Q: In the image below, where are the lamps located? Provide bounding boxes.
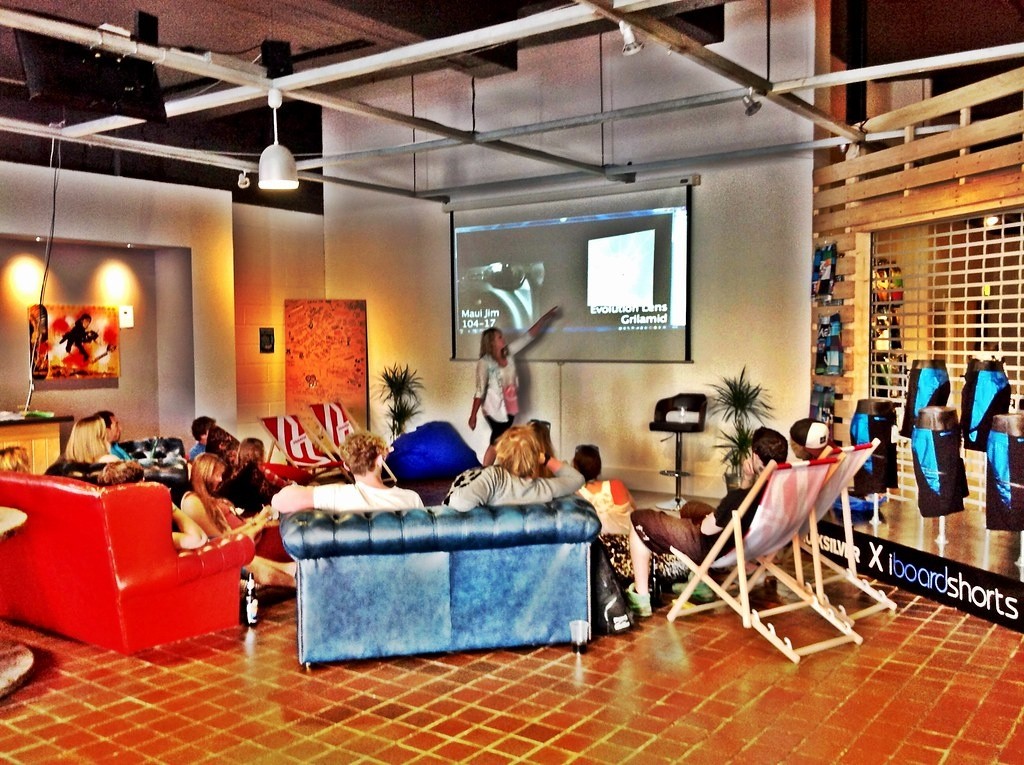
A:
[742,86,761,117]
[258,88,299,190]
[617,20,643,57]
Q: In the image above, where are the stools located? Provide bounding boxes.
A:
[649,392,708,511]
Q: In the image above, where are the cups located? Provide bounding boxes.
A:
[678,406,687,424]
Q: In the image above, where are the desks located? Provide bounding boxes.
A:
[0,505,35,697]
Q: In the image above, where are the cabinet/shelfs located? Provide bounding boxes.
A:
[0,415,75,476]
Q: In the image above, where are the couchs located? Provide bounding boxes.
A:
[280,494,602,671]
[0,470,255,657]
[117,437,190,511]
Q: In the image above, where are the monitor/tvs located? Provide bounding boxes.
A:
[10,7,169,126]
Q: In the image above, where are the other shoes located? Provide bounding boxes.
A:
[671,570,714,602]
[623,583,653,617]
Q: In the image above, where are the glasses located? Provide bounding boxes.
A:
[528,419,551,430]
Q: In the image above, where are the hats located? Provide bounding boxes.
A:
[789,418,840,457]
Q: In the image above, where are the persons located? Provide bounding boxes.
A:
[574,445,635,535]
[443,419,586,512]
[0,446,31,474]
[182,417,296,588]
[749,418,843,609]
[466,304,560,467]
[44,410,131,486]
[100,459,207,549]
[271,433,426,511]
[628,426,788,616]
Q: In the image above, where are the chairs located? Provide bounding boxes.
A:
[260,415,355,484]
[667,437,897,664]
[310,403,398,483]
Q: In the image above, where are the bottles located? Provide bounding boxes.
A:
[650,555,662,607]
[243,572,259,627]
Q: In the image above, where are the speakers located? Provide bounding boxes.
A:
[261,40,294,79]
[136,9,160,46]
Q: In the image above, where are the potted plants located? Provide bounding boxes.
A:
[706,365,776,495]
